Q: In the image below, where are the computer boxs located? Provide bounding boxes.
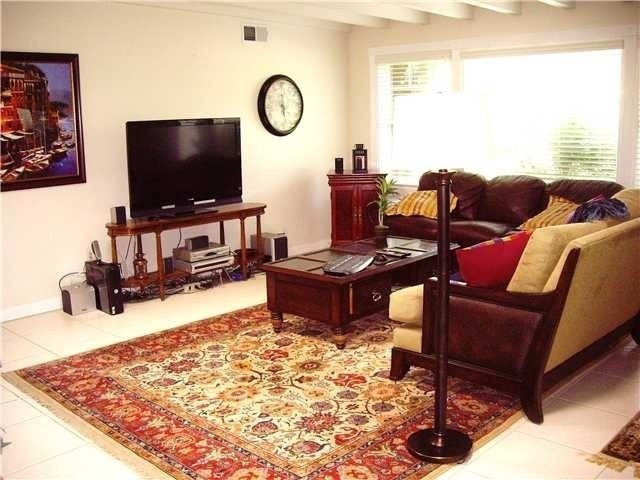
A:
[85,260,124,315]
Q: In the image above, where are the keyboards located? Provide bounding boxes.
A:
[323,254,375,276]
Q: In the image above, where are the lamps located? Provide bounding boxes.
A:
[390,93,489,464]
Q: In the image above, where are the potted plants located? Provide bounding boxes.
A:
[365,175,401,243]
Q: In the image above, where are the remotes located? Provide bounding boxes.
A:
[384,248,411,256]
[376,250,405,258]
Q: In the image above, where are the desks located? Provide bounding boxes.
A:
[105,204,267,300]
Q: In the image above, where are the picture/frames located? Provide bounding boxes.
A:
[0,51,86,191]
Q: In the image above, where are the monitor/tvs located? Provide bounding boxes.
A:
[126,117,243,218]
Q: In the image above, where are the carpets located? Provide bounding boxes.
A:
[586,409,640,480]
[0,304,632,479]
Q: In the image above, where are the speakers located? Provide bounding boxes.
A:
[91,240,102,264]
[249,231,288,262]
[111,207,126,224]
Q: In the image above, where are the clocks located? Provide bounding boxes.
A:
[257,75,303,136]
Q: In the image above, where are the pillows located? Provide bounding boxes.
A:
[604,187,640,227]
[543,179,624,210]
[419,170,487,220]
[482,175,549,225]
[516,195,580,232]
[455,230,531,286]
[387,189,458,219]
[506,221,608,295]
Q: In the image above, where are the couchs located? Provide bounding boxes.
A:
[390,217,640,425]
[383,211,549,248]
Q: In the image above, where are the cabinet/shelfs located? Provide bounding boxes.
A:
[327,174,388,249]
